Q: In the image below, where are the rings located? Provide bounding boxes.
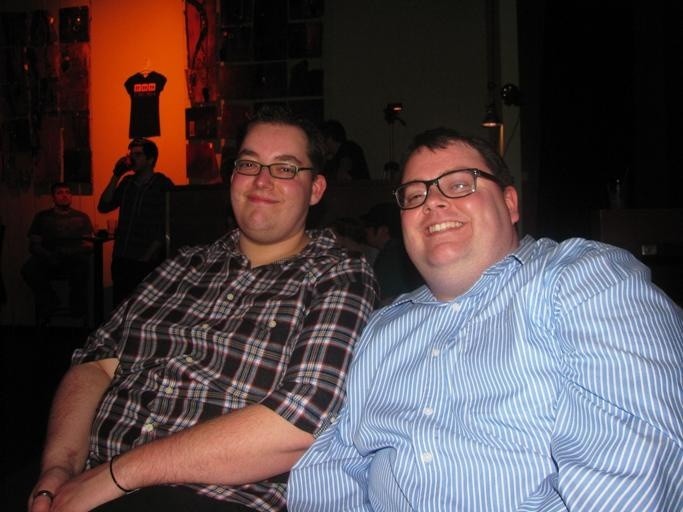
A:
[33,489,53,500]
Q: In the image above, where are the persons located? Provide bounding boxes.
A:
[20,182,92,307]
[316,121,423,306]
[96,138,173,307]
[286,126,682,511]
[27,106,381,511]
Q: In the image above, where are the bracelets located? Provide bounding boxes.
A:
[109,455,131,492]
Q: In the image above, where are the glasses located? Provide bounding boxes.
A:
[392,169,496,210]
[235,160,312,179]
